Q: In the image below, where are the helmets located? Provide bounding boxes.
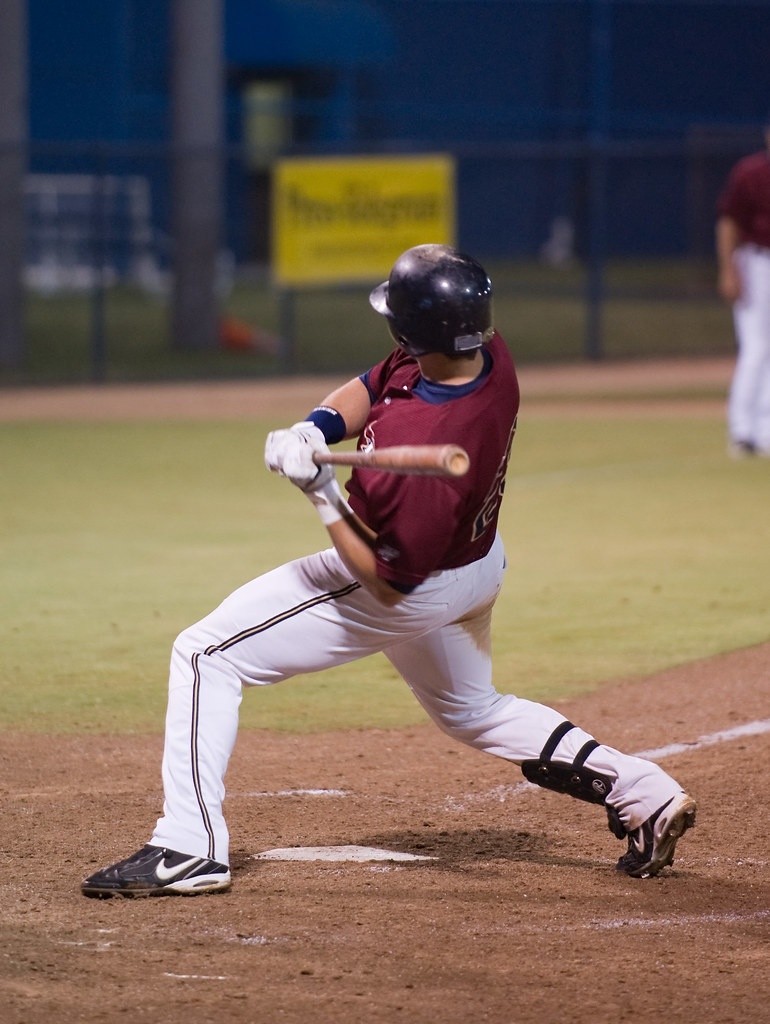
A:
[369,243,495,352]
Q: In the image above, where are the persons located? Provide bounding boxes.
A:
[80,245,700,897]
[718,126,770,460]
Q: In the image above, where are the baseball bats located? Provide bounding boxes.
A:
[313,442,472,478]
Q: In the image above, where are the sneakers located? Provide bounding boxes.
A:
[81,845,231,898]
[617,792,698,880]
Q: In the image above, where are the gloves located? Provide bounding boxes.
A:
[263,420,334,494]
